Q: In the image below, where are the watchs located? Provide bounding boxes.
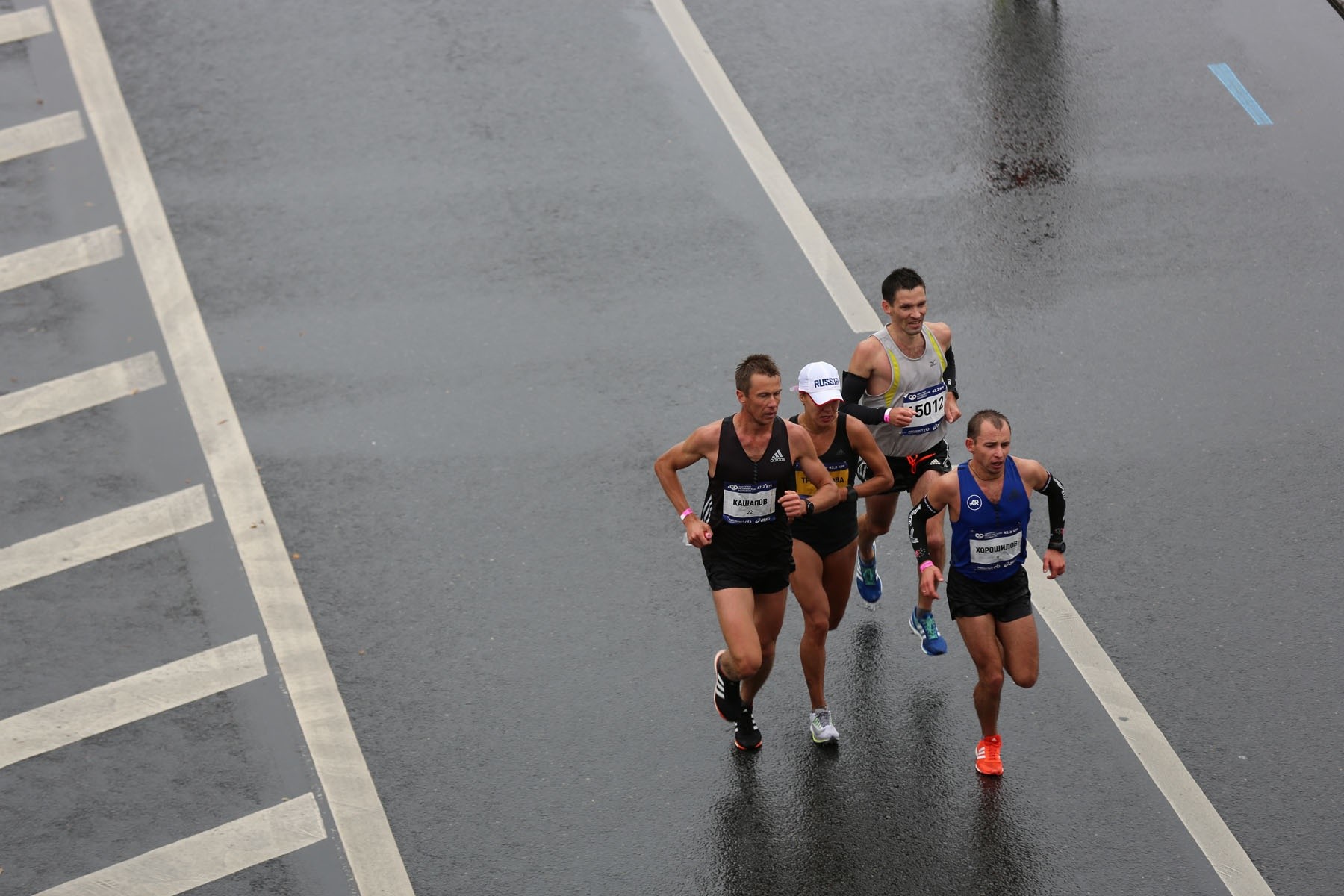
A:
[846,485,858,503]
[1047,542,1066,553]
[801,498,814,515]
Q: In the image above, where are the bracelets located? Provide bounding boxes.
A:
[884,407,892,424]
[919,561,934,574]
[680,508,692,521]
[948,387,959,400]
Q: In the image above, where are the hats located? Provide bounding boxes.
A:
[798,361,845,406]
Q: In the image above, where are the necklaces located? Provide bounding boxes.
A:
[967,461,1004,480]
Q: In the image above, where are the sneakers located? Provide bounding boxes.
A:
[809,705,840,744]
[908,606,947,656]
[733,700,762,750]
[975,735,1004,775]
[713,649,743,723]
[856,540,882,603]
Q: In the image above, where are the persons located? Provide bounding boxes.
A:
[837,266,962,657]
[786,362,895,744]
[908,408,1068,775]
[654,355,841,751]
